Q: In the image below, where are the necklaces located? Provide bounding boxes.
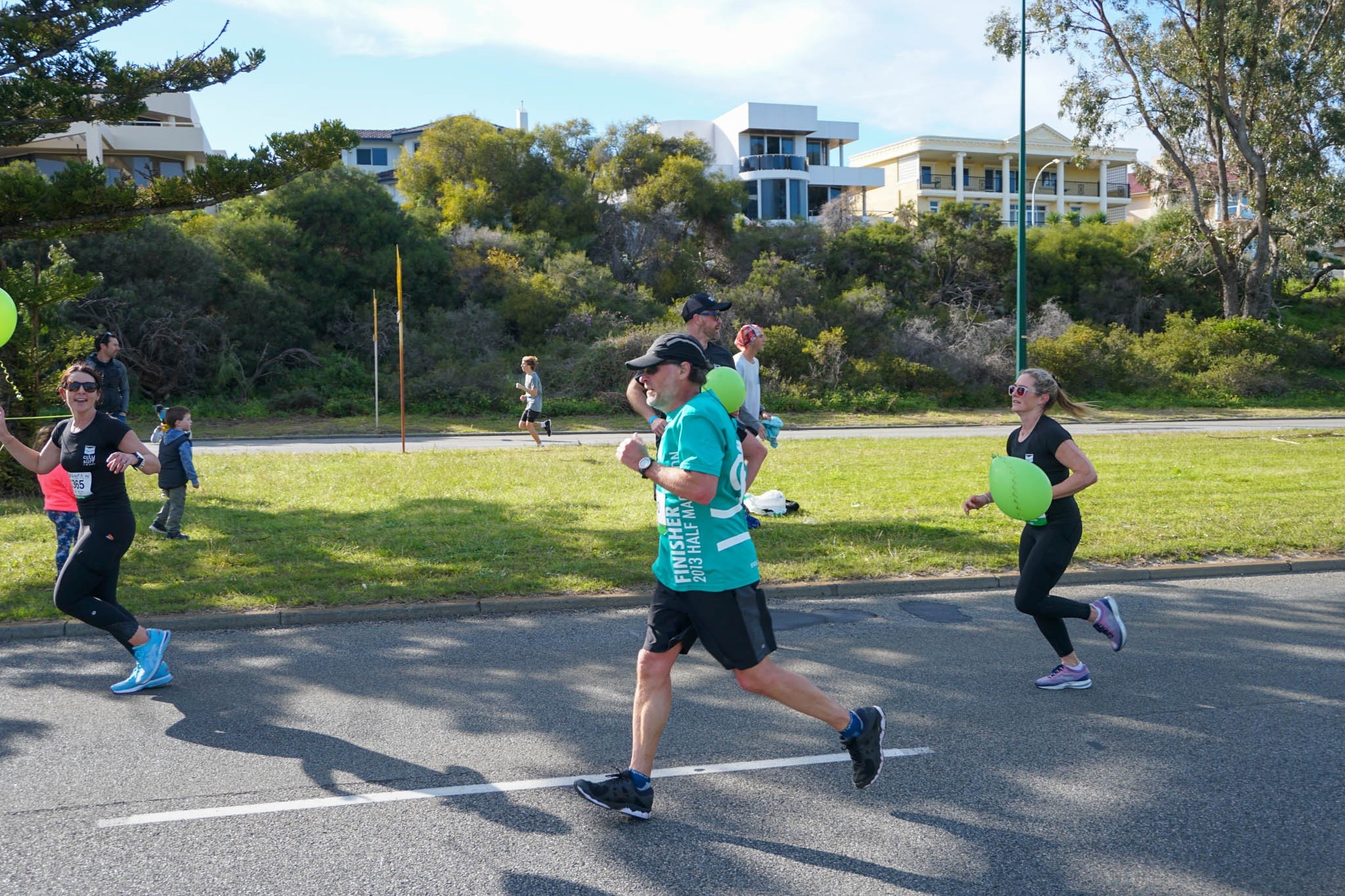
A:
[1021,414,1040,436]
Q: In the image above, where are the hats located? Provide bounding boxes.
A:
[733,323,764,351]
[625,332,714,371]
[682,293,733,323]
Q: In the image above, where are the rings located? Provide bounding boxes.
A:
[122,460,125,463]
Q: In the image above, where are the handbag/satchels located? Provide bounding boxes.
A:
[743,489,787,517]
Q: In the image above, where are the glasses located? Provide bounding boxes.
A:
[99,332,112,343]
[63,381,100,393]
[1008,385,1042,397]
[642,360,680,374]
[699,311,723,321]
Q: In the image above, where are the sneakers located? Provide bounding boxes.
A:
[572,764,654,820]
[109,660,174,694]
[543,419,552,437]
[131,628,171,685]
[166,532,190,540]
[148,521,168,534]
[838,705,888,791]
[741,503,761,529]
[1034,661,1092,690]
[1089,596,1128,652]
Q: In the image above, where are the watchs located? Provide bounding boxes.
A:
[638,456,655,479]
[131,451,146,471]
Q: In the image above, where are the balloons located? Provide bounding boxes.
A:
[0,288,17,347]
[701,366,746,414]
[989,453,1053,522]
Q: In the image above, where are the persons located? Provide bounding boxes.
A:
[0,362,173,694]
[573,333,887,820]
[31,424,80,580]
[515,355,552,448]
[625,293,772,531]
[85,331,129,423]
[963,368,1127,691]
[148,404,199,541]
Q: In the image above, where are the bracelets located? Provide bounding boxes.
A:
[982,494,990,507]
[648,415,658,426]
[3,436,15,445]
